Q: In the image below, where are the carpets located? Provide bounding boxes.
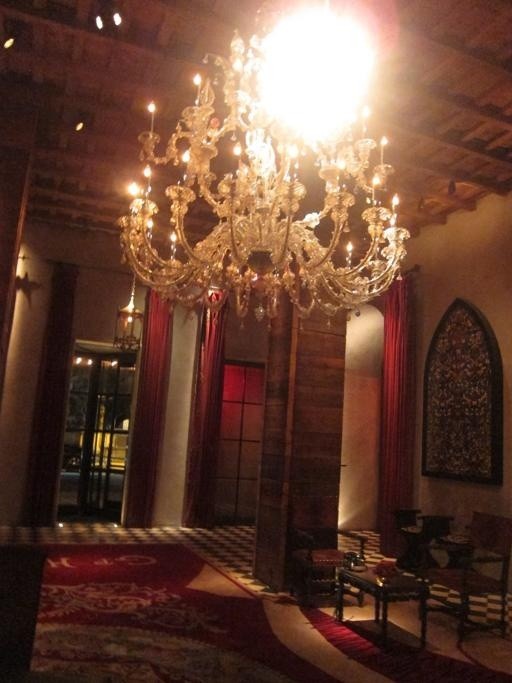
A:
[1,541,512,682]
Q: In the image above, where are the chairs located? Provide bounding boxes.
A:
[289,494,368,609]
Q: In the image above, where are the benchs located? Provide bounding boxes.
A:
[398,511,512,647]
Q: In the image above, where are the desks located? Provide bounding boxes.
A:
[337,565,429,648]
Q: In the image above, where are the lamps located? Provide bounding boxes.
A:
[117,1,410,335]
